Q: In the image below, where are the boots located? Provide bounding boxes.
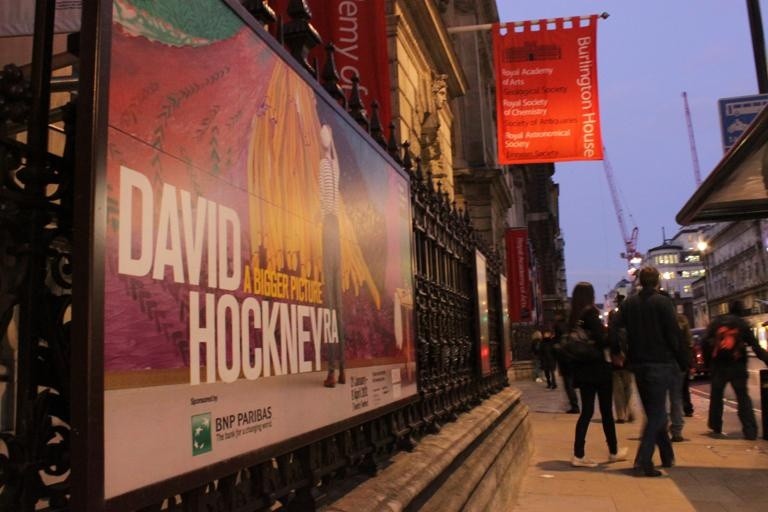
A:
[324,368,345,387]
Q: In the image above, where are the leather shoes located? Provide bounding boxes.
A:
[566,408,580,413]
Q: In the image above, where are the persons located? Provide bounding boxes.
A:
[532,328,559,388]
[699,301,767,440]
[313,121,348,389]
[608,267,694,472]
[553,314,580,413]
[569,282,628,467]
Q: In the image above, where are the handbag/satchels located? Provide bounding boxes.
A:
[551,323,591,365]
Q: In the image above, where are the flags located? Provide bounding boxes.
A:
[491,14,606,163]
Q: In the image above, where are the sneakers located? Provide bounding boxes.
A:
[635,460,675,476]
[608,447,628,461]
[570,456,598,467]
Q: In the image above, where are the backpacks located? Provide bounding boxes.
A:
[712,315,744,369]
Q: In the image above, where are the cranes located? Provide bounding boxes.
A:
[601,146,642,264]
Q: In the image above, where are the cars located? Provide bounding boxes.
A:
[689,327,710,380]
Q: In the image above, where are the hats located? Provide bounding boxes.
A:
[319,122,331,148]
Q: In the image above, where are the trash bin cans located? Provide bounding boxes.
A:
[758,369,767,441]
